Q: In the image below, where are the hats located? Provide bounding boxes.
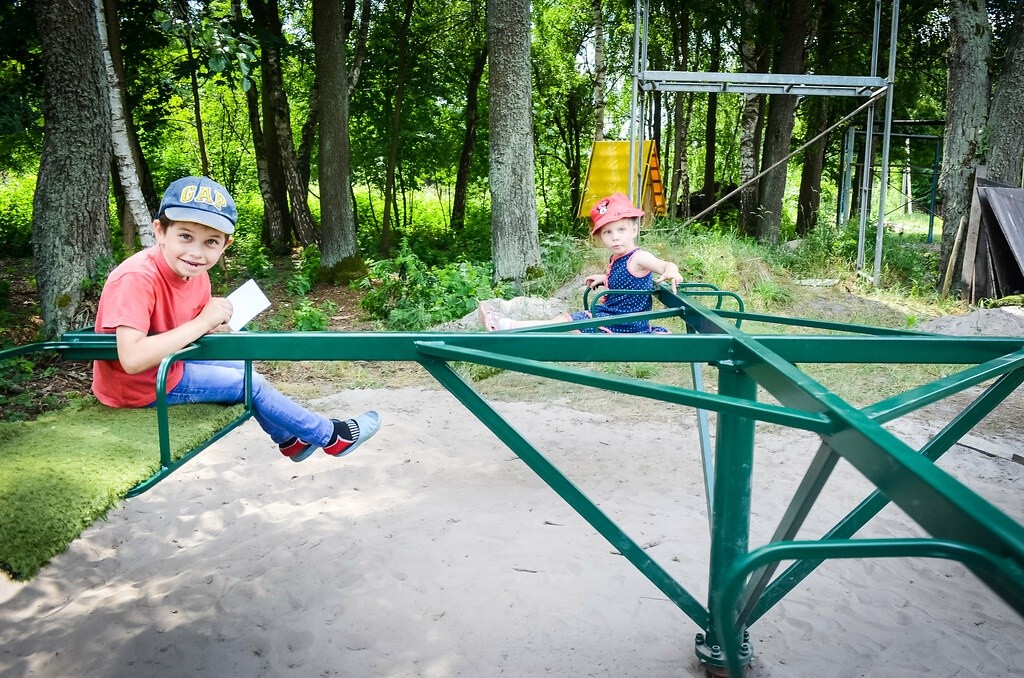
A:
[158,176,238,234]
[590,193,645,236]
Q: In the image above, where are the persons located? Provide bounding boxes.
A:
[478,191,682,335]
[91,176,381,462]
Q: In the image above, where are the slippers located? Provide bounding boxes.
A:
[289,419,340,463]
[333,411,381,458]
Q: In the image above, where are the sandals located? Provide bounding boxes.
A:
[478,306,501,332]
